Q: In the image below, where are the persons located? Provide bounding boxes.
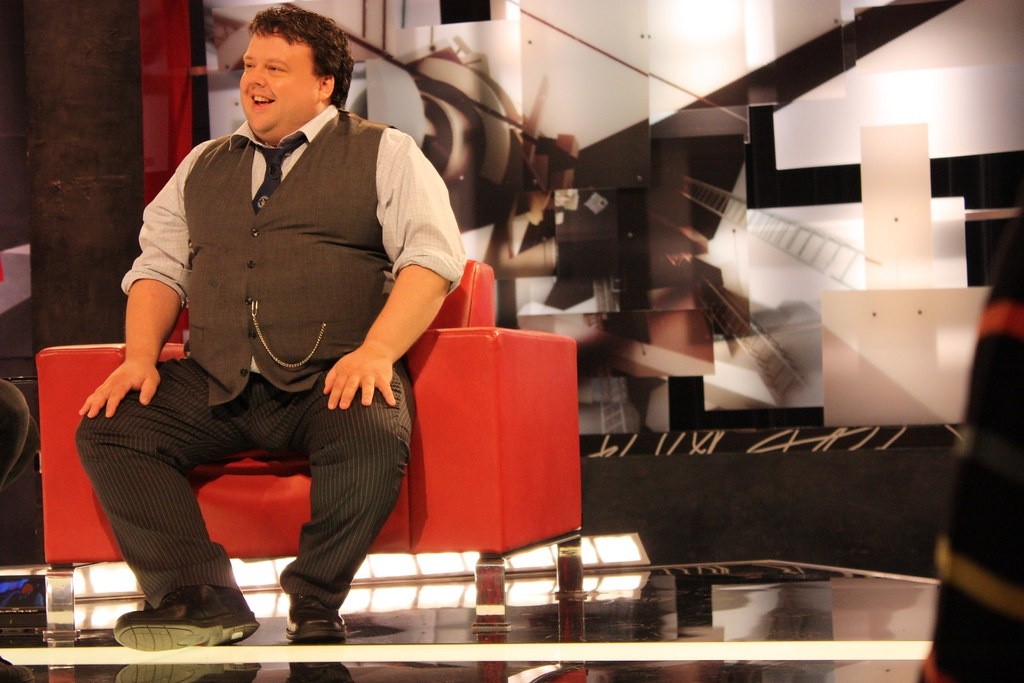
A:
[0,378,40,493]
[0,581,44,607]
[76,8,465,652]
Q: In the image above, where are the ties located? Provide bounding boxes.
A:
[249,133,311,216]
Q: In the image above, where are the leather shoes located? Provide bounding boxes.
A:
[285,586,347,642]
[113,582,261,652]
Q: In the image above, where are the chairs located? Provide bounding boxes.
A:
[35,257,583,639]
